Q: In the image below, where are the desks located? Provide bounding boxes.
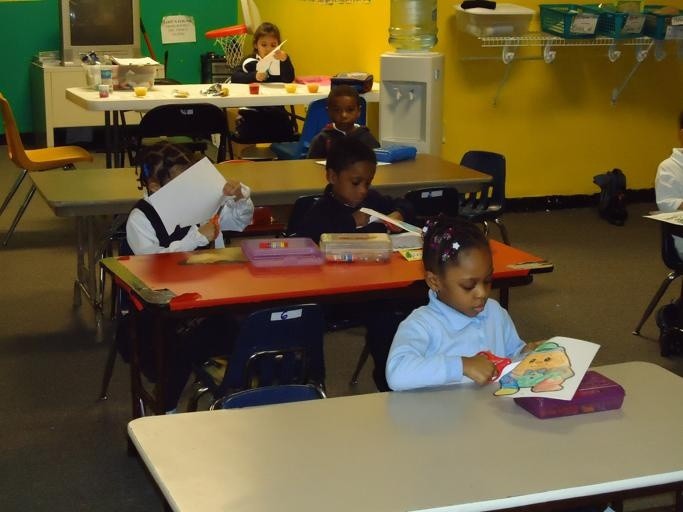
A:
[126,361,682,512]
[26,149,496,342]
[64,82,380,171]
[97,238,554,455]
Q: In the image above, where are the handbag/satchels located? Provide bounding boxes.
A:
[596,167,629,226]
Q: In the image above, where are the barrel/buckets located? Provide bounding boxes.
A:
[389,0,438,49]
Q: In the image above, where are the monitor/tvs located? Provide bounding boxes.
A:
[62,1,141,61]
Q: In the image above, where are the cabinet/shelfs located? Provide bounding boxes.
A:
[29,59,167,149]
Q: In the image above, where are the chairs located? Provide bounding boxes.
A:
[199,52,300,162]
[400,185,460,230]
[630,222,682,337]
[297,97,366,160]
[0,94,93,250]
[118,104,229,168]
[458,150,510,246]
[222,205,286,247]
[365,294,430,393]
[207,347,330,411]
[286,192,368,332]
[99,217,147,418]
[185,300,325,411]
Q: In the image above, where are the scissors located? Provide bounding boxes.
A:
[209,200,227,239]
[266,61,272,80]
[475,351,531,384]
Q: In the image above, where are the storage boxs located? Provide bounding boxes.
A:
[452,1,536,38]
[643,5,683,42]
[538,4,608,39]
[578,5,652,40]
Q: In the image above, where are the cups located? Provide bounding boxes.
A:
[248,84,259,95]
[132,86,149,97]
[305,84,320,93]
[98,84,113,98]
[284,84,297,94]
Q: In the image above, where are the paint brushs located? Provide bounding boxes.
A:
[259,241,289,248]
[337,235,377,239]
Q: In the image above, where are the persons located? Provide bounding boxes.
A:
[293,141,406,394]
[655,114,683,358]
[308,84,380,162]
[114,143,256,418]
[387,213,546,392]
[235,23,299,147]
